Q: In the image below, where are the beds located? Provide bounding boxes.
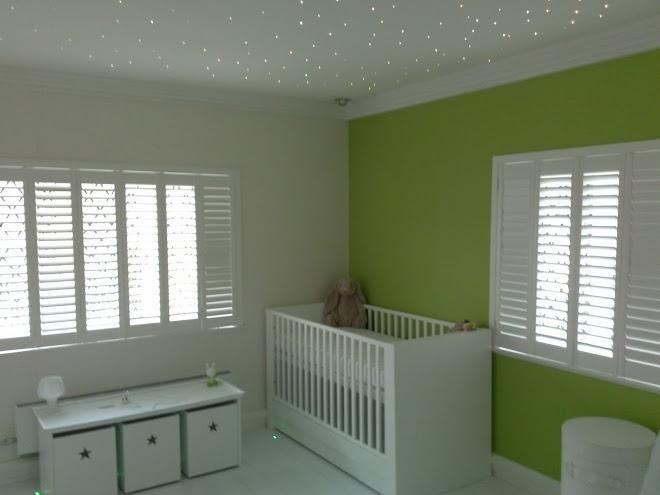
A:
[264,302,493,495]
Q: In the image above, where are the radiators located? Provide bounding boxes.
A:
[15,371,234,457]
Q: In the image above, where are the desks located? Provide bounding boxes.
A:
[32,370,246,495]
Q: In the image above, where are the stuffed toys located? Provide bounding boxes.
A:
[325,277,367,328]
